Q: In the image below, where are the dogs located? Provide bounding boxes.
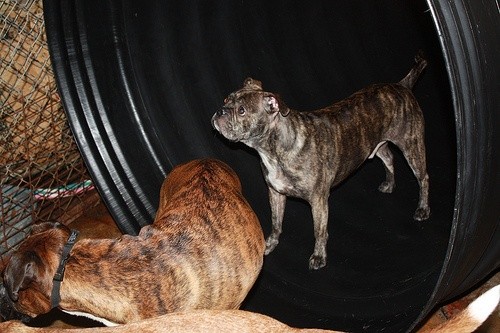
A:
[209,50,432,270]
[0,156,264,326]
[0,283,499,333]
